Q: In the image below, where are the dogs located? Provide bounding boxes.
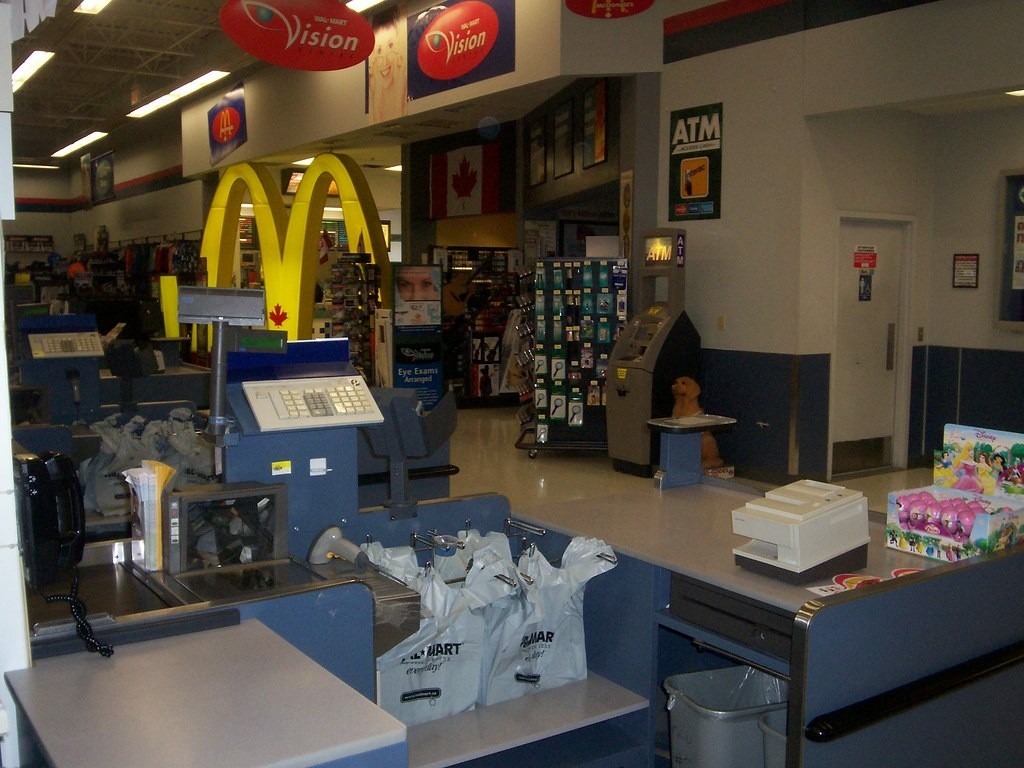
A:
[670,376,724,467]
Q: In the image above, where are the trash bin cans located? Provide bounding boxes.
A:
[664,664,788,768]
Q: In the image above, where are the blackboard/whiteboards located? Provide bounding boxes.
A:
[991,167,1024,330]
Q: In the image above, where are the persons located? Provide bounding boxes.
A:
[396,266,437,301]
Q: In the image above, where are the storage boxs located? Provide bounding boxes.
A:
[467,304,519,398]
[884,423,1023,563]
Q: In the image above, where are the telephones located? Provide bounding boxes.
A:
[27,450,86,586]
